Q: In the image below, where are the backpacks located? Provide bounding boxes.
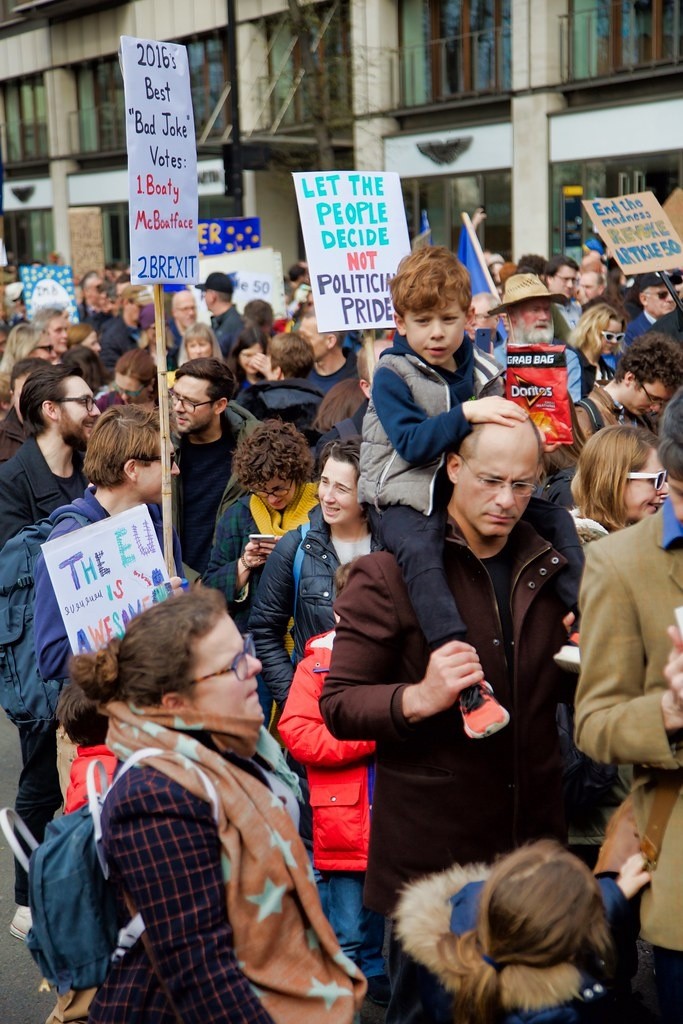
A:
[0,512,94,722]
[0,747,220,996]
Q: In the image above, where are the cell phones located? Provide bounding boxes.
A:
[248,533,275,546]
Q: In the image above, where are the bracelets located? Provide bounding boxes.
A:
[241,554,251,569]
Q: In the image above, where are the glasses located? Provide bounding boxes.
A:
[56,396,98,411]
[168,388,216,414]
[627,469,668,490]
[456,454,537,497]
[643,290,680,299]
[255,475,294,498]
[185,632,257,685]
[33,345,53,354]
[132,452,175,469]
[635,374,669,407]
[600,331,625,343]
[555,274,579,285]
[112,380,145,399]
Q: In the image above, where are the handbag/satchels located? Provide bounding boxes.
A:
[592,793,660,876]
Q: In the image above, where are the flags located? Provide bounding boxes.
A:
[458,226,508,345]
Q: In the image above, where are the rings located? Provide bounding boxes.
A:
[258,555,260,562]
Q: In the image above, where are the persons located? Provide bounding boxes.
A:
[0,246,683,1024]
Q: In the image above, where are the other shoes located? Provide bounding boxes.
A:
[10,914,30,940]
[366,974,392,1006]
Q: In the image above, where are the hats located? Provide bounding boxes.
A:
[195,272,234,294]
[635,271,683,290]
[487,273,568,315]
[5,282,24,300]
[140,303,169,328]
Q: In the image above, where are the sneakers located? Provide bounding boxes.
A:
[553,633,583,673]
[459,680,510,738]
